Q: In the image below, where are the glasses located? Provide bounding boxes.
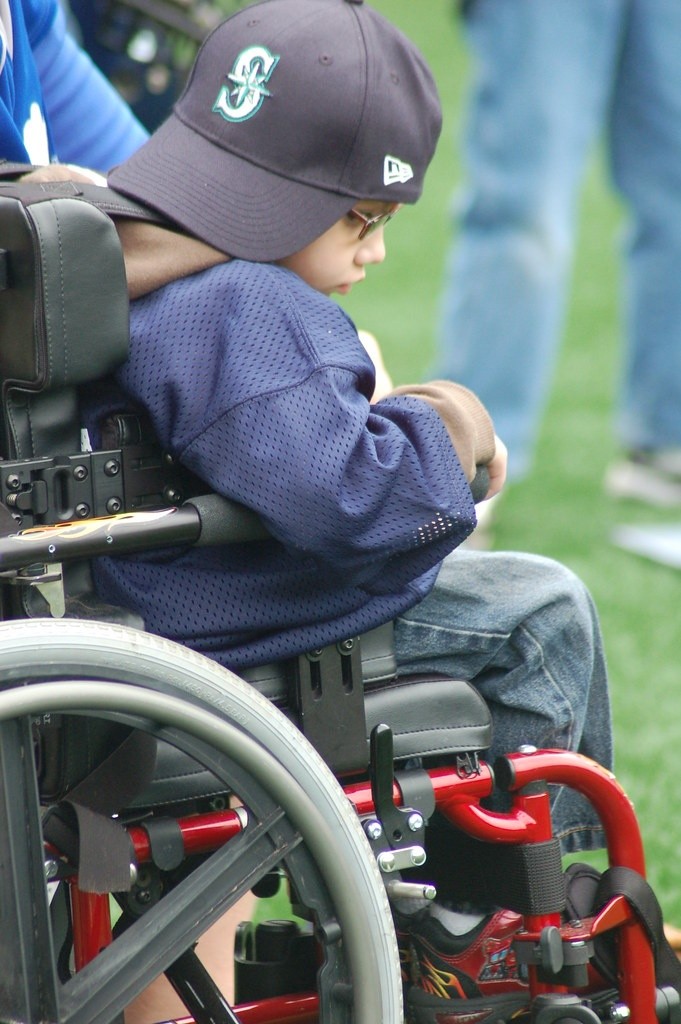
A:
[350,203,402,241]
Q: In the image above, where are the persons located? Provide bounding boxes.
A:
[431,0,681,524]
[0,0,615,1023]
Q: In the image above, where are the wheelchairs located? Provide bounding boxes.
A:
[1,184,681,1023]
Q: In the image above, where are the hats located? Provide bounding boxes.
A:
[106,0,443,262]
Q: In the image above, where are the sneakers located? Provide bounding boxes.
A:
[394,903,622,1024]
